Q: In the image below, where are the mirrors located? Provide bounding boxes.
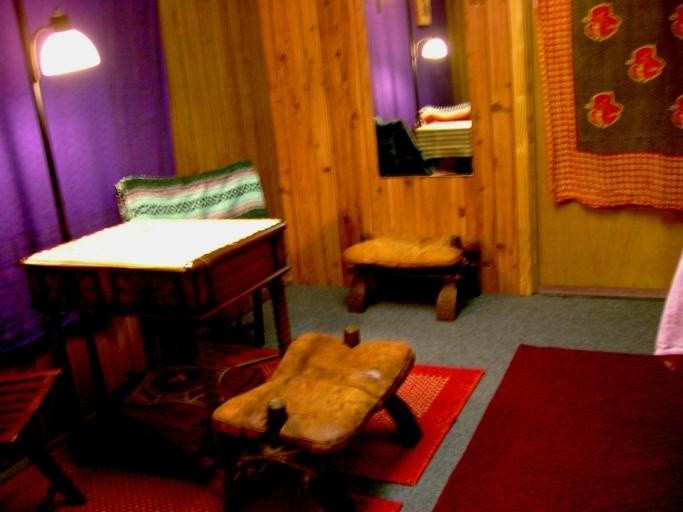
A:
[366,0,471,178]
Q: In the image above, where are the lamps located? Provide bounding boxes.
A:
[404,0,450,124]
[13,0,109,399]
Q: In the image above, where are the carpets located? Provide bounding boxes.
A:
[0,442,404,512]
[431,344,683,512]
[195,342,486,489]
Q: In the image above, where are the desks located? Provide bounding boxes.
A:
[21,216,292,395]
[414,120,472,173]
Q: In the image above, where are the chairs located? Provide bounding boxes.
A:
[113,160,270,347]
[375,115,432,177]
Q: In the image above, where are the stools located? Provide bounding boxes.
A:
[0,368,87,505]
[212,324,424,512]
[341,231,481,321]
